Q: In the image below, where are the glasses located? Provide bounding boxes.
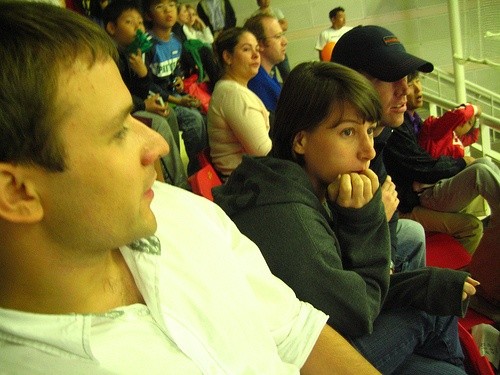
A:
[261,33,286,39]
[149,3,178,11]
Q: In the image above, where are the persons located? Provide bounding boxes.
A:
[413,104,499,212]
[0,0,386,375]
[329,24,434,273]
[242,13,287,112]
[315,7,355,62]
[211,61,480,375]
[248,0,291,85]
[383,68,485,257]
[88,1,237,191]
[206,27,272,185]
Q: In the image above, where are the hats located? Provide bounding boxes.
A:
[329,25,434,83]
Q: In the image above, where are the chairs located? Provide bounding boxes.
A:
[426,231,500,375]
